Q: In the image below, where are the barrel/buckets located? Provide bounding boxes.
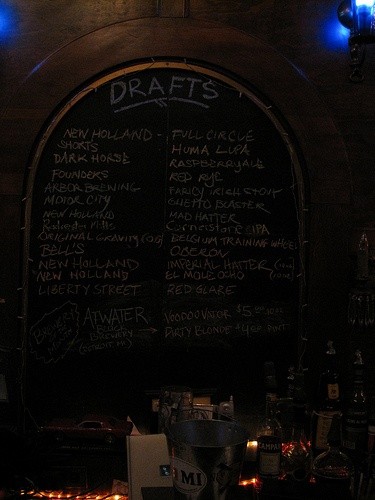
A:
[158,385,193,433]
[164,408,251,500]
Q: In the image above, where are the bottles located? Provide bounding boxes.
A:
[257,392,282,480]
[347,231,375,330]
[283,339,375,500]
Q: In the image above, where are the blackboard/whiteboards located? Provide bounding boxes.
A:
[21,63,305,433]
